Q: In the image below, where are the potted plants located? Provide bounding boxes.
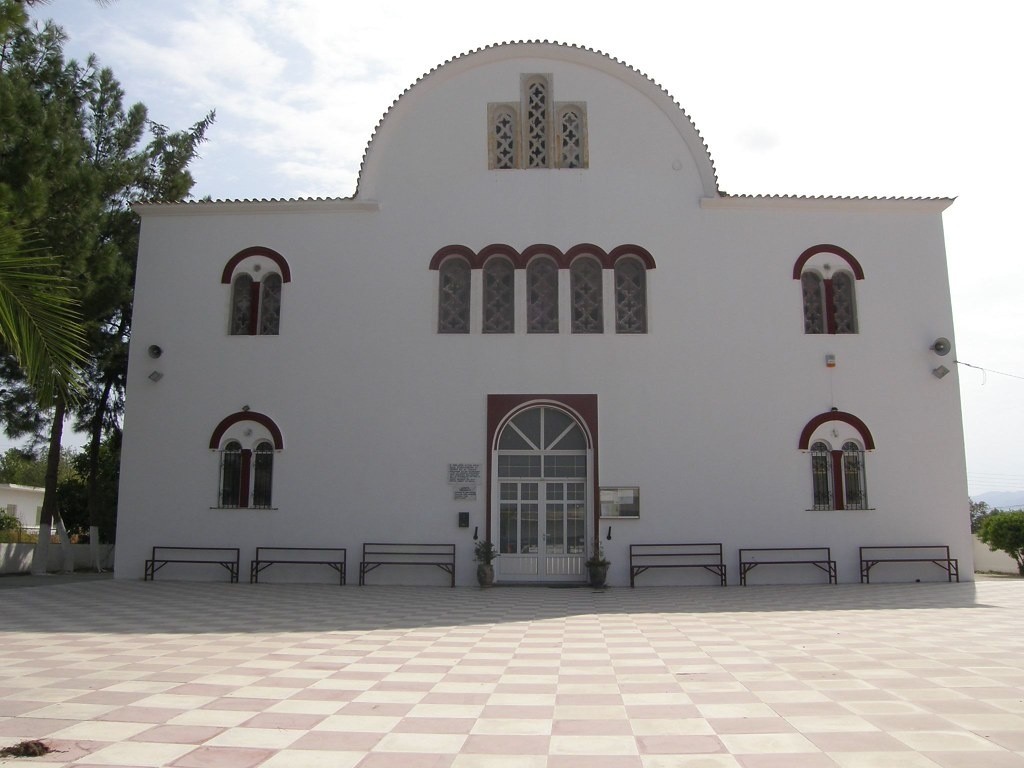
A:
[472,537,501,587]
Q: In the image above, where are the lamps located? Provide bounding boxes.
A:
[932,364,948,378]
[149,371,163,382]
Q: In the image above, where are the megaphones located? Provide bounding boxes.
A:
[148,344,163,359]
[929,337,952,356]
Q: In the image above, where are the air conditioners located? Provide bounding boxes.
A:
[584,536,611,585]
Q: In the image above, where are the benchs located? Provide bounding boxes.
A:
[629,543,727,588]
[859,546,959,583]
[144,546,240,582]
[251,547,346,585]
[739,548,838,586]
[359,542,456,588]
